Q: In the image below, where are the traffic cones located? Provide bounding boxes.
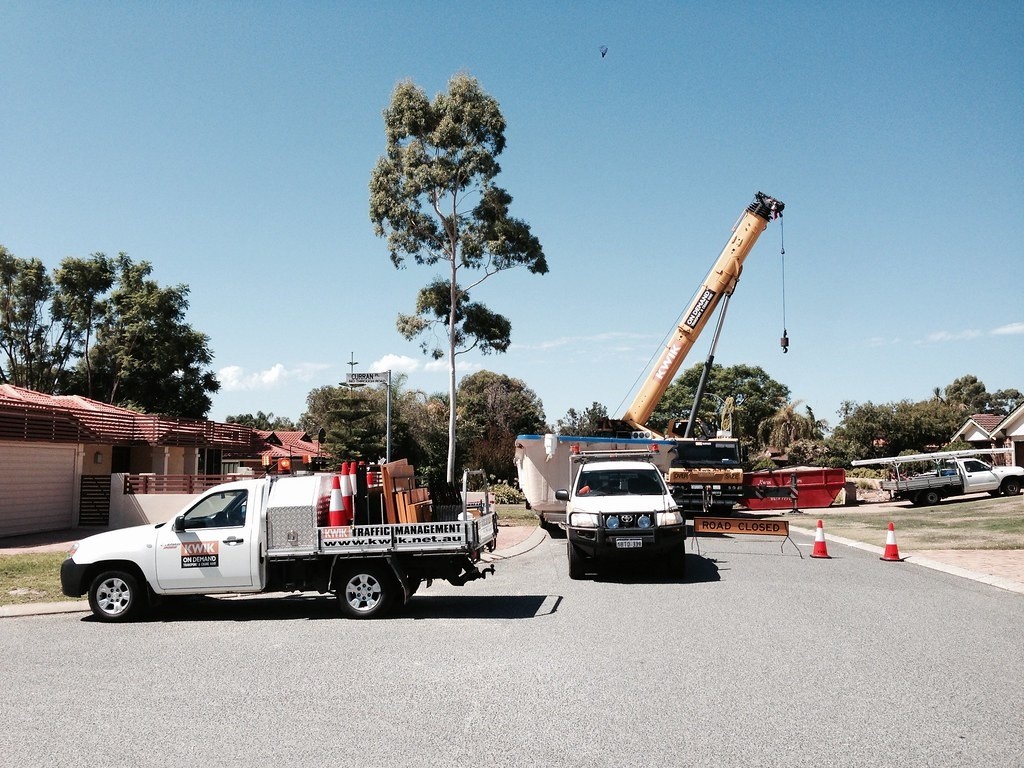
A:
[327,460,373,526]
[809,519,834,559]
[879,521,904,562]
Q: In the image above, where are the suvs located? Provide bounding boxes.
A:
[555,444,689,581]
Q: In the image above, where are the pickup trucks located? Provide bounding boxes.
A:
[880,458,1024,505]
[60,453,500,621]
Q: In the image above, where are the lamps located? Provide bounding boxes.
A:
[94,452,104,464]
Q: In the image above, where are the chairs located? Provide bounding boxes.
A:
[628,478,649,494]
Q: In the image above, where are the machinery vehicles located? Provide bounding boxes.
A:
[592,191,790,529]
